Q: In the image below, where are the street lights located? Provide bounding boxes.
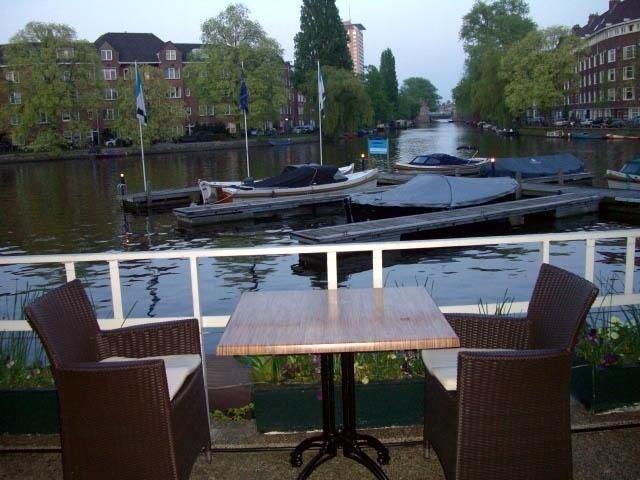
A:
[284,117,289,136]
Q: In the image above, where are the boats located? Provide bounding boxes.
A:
[545,130,640,140]
[448,120,454,123]
[465,119,520,138]
[357,119,416,138]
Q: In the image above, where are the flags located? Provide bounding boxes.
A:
[132,64,149,127]
[236,68,251,113]
[317,72,331,119]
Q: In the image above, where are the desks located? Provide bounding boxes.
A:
[214,283,463,478]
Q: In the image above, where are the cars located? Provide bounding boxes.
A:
[21,142,34,152]
[523,115,640,129]
[0,141,19,153]
[103,135,133,148]
[240,124,316,137]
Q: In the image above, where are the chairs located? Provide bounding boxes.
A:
[22,277,213,480]
[419,264,599,479]
